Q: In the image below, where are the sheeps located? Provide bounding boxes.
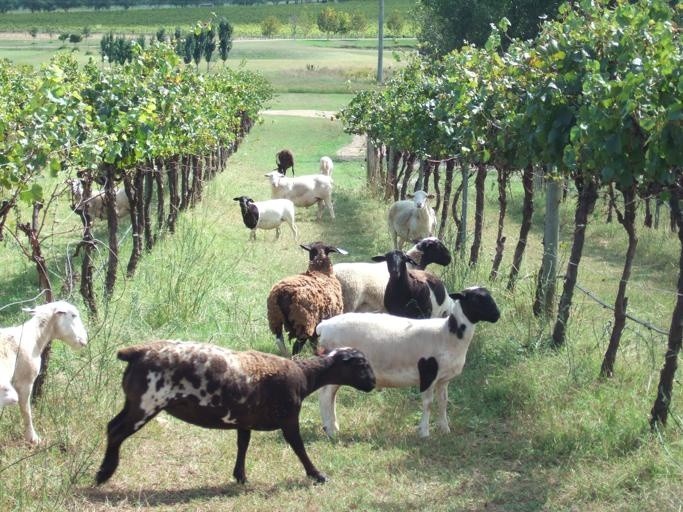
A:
[372,251,455,320]
[266,241,346,358]
[319,156,332,175]
[276,150,294,176]
[316,286,501,444]
[94,340,377,487]
[387,191,440,254]
[264,171,336,220]
[233,195,298,246]
[332,237,453,313]
[0,297,90,454]
[65,178,138,220]
